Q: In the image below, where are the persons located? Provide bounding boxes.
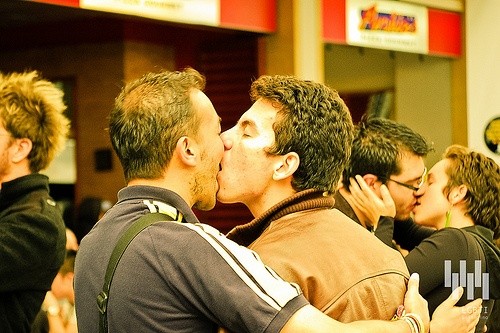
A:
[73,68,430,333]
[348,144,500,333]
[0,70,73,333]
[217,74,411,322]
[330,116,488,333]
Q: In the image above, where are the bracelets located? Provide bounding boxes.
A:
[393,305,424,333]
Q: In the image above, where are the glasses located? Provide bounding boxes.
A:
[378,167,427,191]
